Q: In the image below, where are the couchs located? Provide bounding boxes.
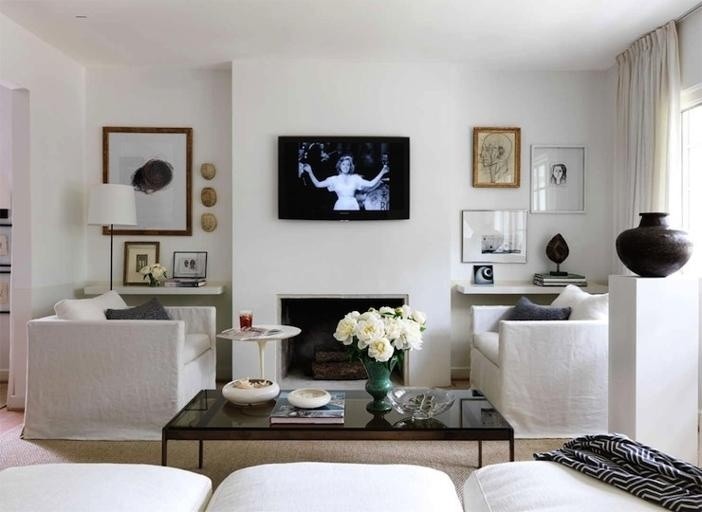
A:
[1,433,701,512]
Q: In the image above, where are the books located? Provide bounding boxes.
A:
[164,280,206,287]
[269,391,345,424]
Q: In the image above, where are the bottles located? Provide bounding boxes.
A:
[617,212,691,277]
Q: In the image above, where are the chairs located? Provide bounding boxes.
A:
[18,290,217,442]
[470,284,609,438]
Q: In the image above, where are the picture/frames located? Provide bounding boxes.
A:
[173,250,208,278]
[461,209,528,265]
[101,126,193,236]
[529,144,588,215]
[472,126,521,188]
[123,241,160,286]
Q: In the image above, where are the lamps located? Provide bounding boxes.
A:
[87,183,138,290]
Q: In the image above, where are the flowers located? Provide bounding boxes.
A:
[332,302,427,364]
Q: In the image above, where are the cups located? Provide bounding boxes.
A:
[239,309,253,328]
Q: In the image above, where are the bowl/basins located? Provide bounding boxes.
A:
[286,387,332,408]
[222,401,276,420]
[218,379,280,406]
[391,418,450,430]
[387,389,456,419]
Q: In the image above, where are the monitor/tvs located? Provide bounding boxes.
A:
[278,136,409,220]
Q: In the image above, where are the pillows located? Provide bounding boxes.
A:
[550,284,609,321]
[105,298,169,320]
[54,290,127,321]
[507,296,572,321]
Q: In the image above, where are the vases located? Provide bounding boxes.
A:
[356,348,399,414]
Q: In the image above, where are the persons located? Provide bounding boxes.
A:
[182,259,198,273]
[297,143,390,211]
